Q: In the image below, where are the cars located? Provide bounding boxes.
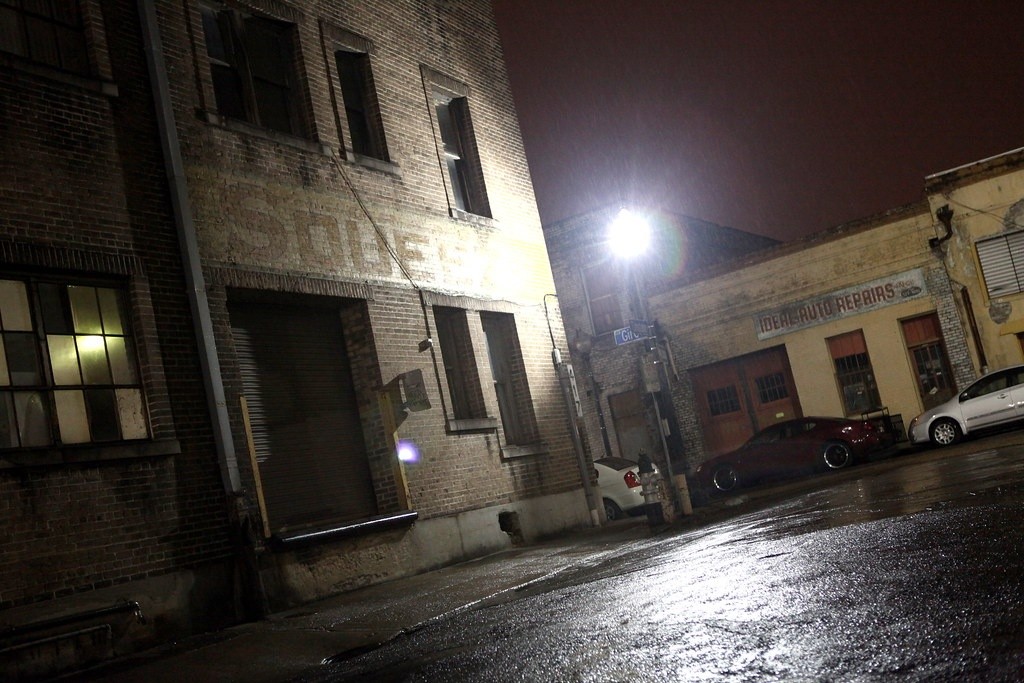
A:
[694,417,878,497]
[908,365,1023,448]
[591,457,667,522]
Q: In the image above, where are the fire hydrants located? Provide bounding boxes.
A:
[636,447,670,529]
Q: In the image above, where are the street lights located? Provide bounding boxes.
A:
[606,210,695,516]
